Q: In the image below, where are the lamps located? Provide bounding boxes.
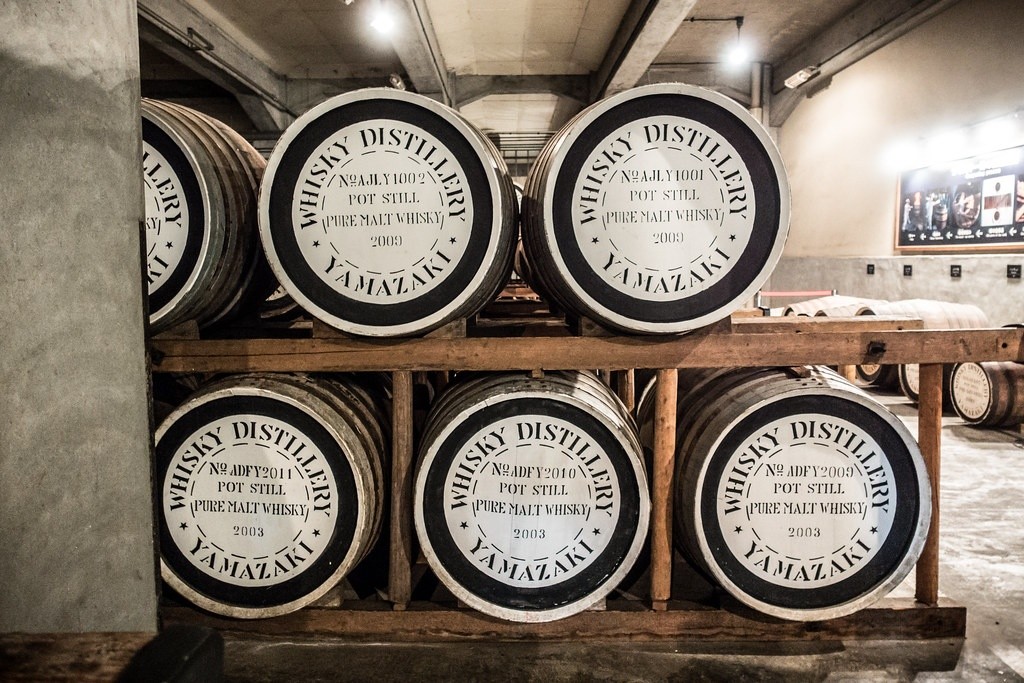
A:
[783,66,821,89]
[389,72,405,91]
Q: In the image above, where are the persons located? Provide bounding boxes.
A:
[902,199,914,231]
[924,196,940,230]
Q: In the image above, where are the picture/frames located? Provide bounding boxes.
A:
[893,144,1024,254]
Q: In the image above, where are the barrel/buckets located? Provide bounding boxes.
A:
[142,82,1024,623]
[935,205,949,231]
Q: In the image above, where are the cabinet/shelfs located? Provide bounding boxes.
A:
[146,283,1024,641]
